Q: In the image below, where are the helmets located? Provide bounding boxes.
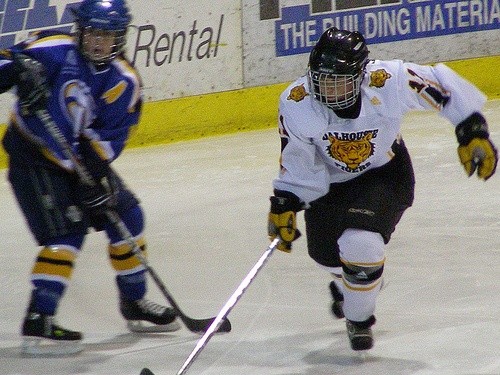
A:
[306,27,370,110]
[73,0,131,64]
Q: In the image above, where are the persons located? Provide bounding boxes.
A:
[0,0,180,354]
[267,25,498,351]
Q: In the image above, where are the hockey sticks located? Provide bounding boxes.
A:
[37,108,231,336]
[140,237,281,374]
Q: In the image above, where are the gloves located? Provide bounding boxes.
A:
[13,58,52,117]
[267,197,302,253]
[455,112,498,181]
[77,179,116,214]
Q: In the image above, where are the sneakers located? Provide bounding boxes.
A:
[120,299,180,332]
[19,313,84,354]
[346,315,376,351]
[329,281,345,318]
